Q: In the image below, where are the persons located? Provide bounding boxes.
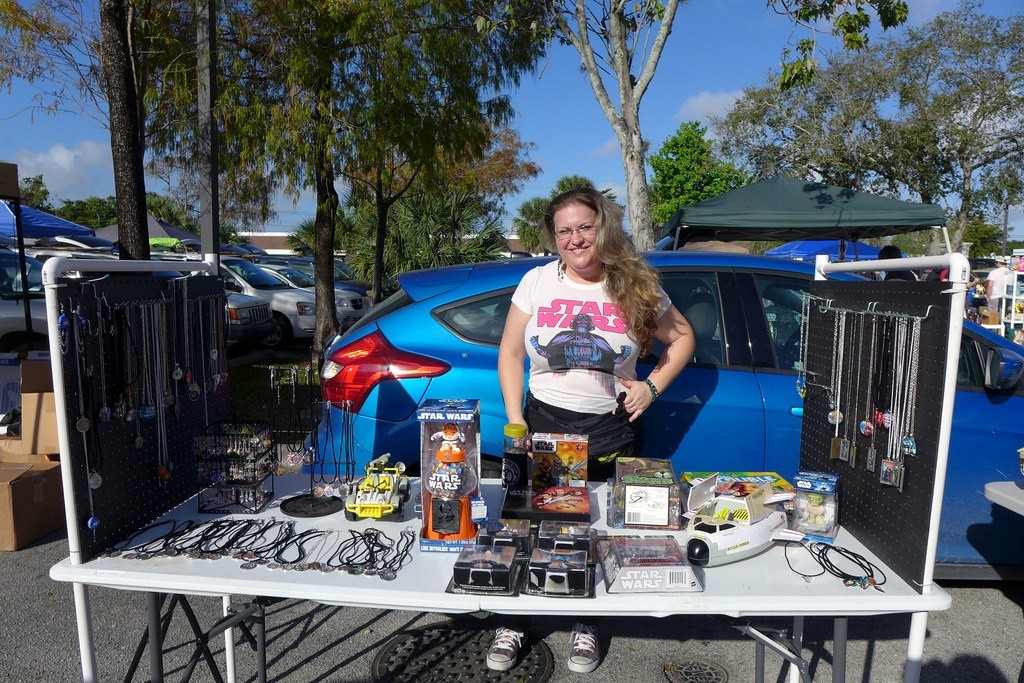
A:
[879,245,917,282]
[485,187,694,673]
[975,283,985,294]
[986,256,1010,312]
[921,268,980,288]
[431,423,465,452]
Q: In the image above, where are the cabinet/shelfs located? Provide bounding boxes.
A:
[981,325,1005,338]
[193,420,275,514]
[1001,272,1024,347]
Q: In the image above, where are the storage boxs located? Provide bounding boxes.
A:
[793,470,840,544]
[680,471,795,511]
[20,360,60,455]
[606,457,680,531]
[528,520,598,565]
[419,398,482,552]
[476,518,538,559]
[0,461,66,552]
[499,484,591,523]
[0,436,51,461]
[532,432,589,491]
[519,548,596,599]
[445,544,521,595]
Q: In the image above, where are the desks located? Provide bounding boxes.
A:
[49,478,952,683]
[984,480,1024,517]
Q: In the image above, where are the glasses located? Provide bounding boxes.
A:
[555,226,597,239]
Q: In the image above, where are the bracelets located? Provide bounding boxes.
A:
[644,379,659,402]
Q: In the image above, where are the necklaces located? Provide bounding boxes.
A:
[104,516,416,580]
[273,365,313,477]
[810,542,887,593]
[313,400,355,498]
[785,541,825,583]
[797,296,921,492]
[56,277,228,542]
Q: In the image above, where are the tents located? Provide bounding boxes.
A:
[0,199,95,237]
[661,176,952,254]
[95,215,201,243]
[764,241,907,262]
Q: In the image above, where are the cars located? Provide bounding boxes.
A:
[0,251,65,356]
[968,258,999,304]
[10,249,277,353]
[297,250,1023,585]
[142,246,386,342]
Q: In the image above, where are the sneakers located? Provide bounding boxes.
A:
[486,625,527,670]
[566,622,602,674]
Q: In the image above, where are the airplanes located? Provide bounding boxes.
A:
[681,473,805,567]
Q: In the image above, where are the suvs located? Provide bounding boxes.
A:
[344,453,410,520]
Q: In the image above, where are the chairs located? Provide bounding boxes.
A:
[681,291,718,365]
